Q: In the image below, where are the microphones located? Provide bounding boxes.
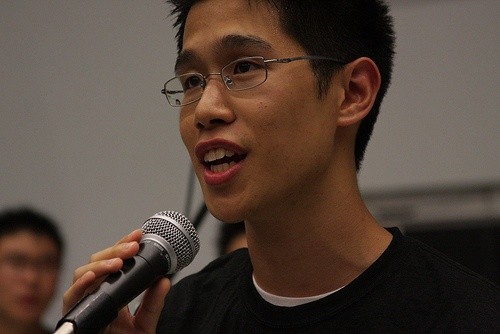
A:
[51,210,200,334]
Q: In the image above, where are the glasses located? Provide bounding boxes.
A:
[160,53,345,109]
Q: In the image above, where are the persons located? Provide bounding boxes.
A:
[216,221,250,259]
[0,207,66,334]
[60,1,500,334]
